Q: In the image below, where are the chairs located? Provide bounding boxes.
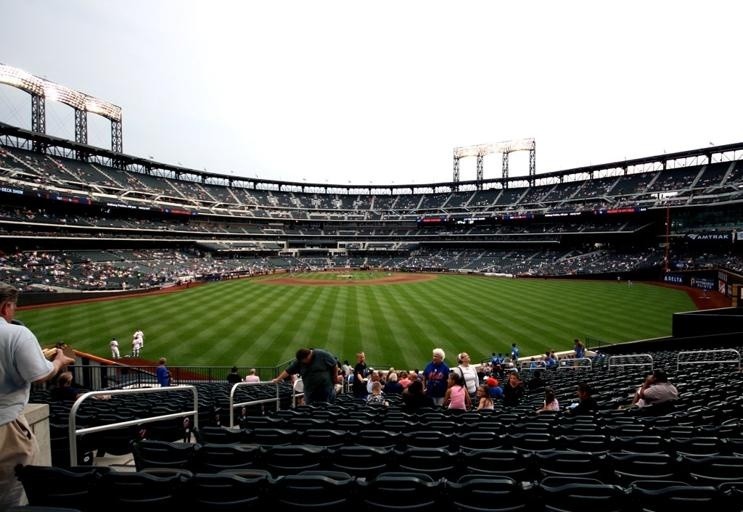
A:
[0,348,742,511]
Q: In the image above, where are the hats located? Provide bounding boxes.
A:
[487,377,498,386]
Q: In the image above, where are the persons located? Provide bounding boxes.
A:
[0,282,76,511]
[50,372,108,403]
[109,329,145,359]
[536,381,600,414]
[155,357,173,385]
[480,343,519,378]
[228,366,260,385]
[530,351,558,373]
[527,371,546,390]
[1,150,743,293]
[45,340,69,388]
[632,370,678,409]
[273,348,523,411]
[574,338,585,358]
